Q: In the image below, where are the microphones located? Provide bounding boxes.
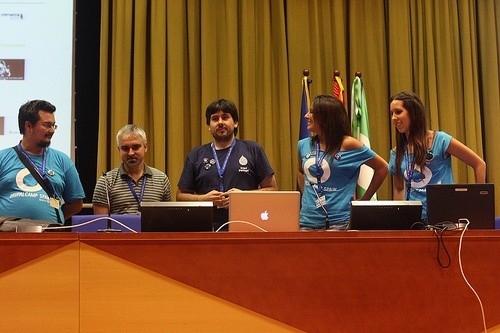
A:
[310,182,338,231]
[97,171,122,232]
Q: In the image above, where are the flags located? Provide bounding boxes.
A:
[332,69,345,109]
[300,70,314,140]
[351,71,379,200]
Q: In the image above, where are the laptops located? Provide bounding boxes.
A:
[229,191,301,232]
[426,183,495,231]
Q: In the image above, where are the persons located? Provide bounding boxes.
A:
[176,99,278,232]
[297,95,389,231]
[388,90,487,229]
[91,124,173,215]
[0,100,88,232]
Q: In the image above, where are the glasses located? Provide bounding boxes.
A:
[36,122,57,129]
